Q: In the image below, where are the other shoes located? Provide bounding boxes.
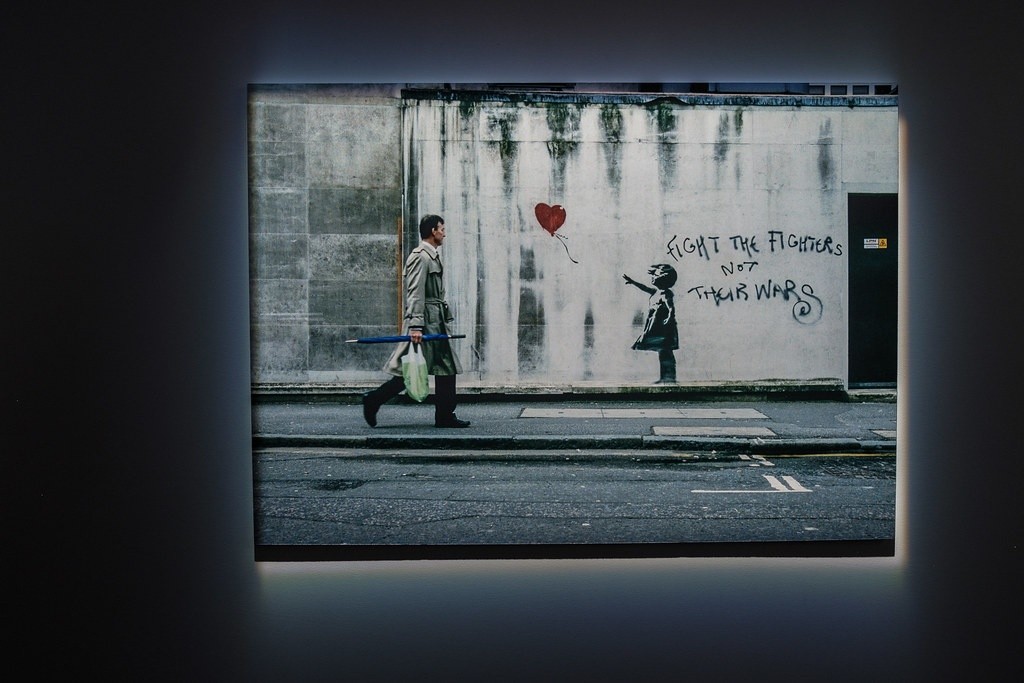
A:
[435,412,470,427]
[362,390,382,426]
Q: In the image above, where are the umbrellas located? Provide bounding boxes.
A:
[345,332,467,340]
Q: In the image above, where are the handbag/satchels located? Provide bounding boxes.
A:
[400,341,429,402]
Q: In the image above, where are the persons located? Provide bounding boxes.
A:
[361,214,470,427]
